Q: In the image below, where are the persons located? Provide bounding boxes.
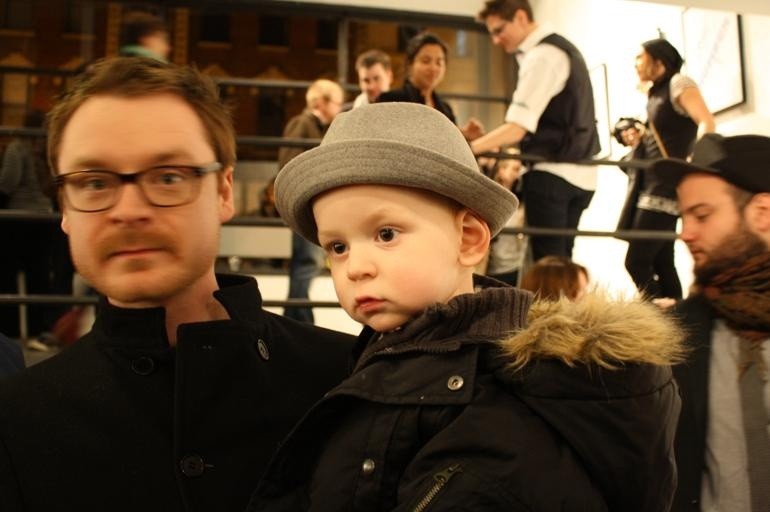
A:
[117,12,171,64]
[246,102,695,511]
[0,107,55,212]
[613,37,719,305]
[278,1,604,321]
[0,57,359,512]
[657,132,769,512]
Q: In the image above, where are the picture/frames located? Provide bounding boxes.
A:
[680,5,745,117]
[586,62,612,161]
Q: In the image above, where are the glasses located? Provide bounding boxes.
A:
[47,162,222,211]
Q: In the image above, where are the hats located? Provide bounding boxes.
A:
[647,134,769,193]
[272,103,519,246]
[643,39,683,72]
